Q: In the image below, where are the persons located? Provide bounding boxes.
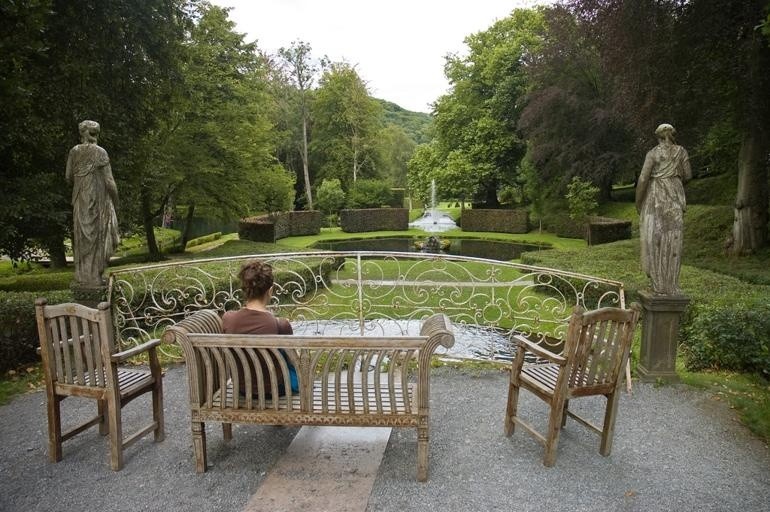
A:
[220,261,309,428]
[634,123,693,295]
[64,120,121,289]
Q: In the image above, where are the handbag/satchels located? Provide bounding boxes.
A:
[285,358,311,392]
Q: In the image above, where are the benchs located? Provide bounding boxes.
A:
[161,308,455,484]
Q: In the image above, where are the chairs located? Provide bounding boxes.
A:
[34,296,164,471]
[504,301,643,468]
